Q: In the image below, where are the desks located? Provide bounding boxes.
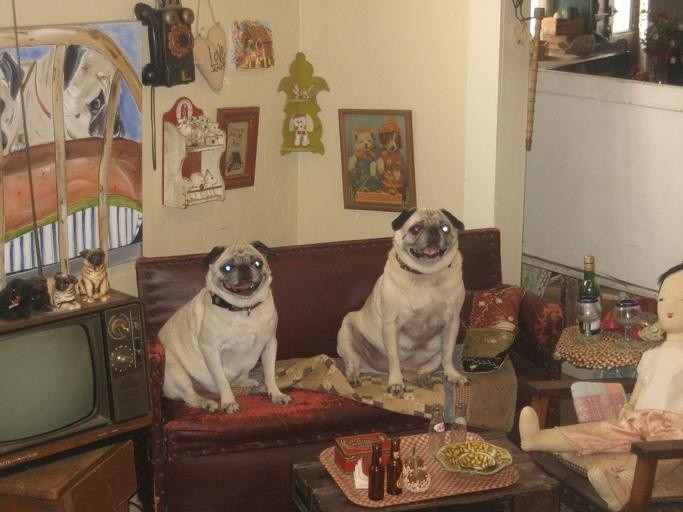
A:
[538,47,631,70]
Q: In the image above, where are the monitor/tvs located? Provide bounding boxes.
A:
[0,288,155,470]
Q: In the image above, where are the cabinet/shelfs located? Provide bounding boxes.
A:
[0,442,139,512]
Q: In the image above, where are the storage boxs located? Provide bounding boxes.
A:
[541,16,585,37]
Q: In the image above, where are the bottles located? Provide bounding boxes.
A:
[451,401,467,444]
[429,404,446,456]
[387,437,403,495]
[577,256,602,335]
[368,444,384,501]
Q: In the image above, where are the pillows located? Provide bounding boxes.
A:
[468,284,525,327]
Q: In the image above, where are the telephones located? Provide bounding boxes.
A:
[134,1,196,171]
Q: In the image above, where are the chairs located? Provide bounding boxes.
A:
[524,376,683,511]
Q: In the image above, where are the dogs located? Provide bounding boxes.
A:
[0,275,53,322]
[157,241,294,414]
[337,207,472,395]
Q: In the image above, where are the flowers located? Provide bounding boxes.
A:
[636,8,683,53]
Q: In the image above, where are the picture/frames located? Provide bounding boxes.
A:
[338,109,417,212]
[216,106,259,190]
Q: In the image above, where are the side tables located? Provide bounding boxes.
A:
[551,325,638,380]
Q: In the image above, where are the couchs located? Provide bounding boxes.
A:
[135,229,564,511]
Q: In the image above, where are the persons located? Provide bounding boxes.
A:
[519,264,683,512]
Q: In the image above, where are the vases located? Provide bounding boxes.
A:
[647,53,669,83]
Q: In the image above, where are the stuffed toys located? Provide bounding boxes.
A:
[0,278,31,320]
[26,277,53,313]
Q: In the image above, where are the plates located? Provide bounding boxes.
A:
[434,440,512,476]
